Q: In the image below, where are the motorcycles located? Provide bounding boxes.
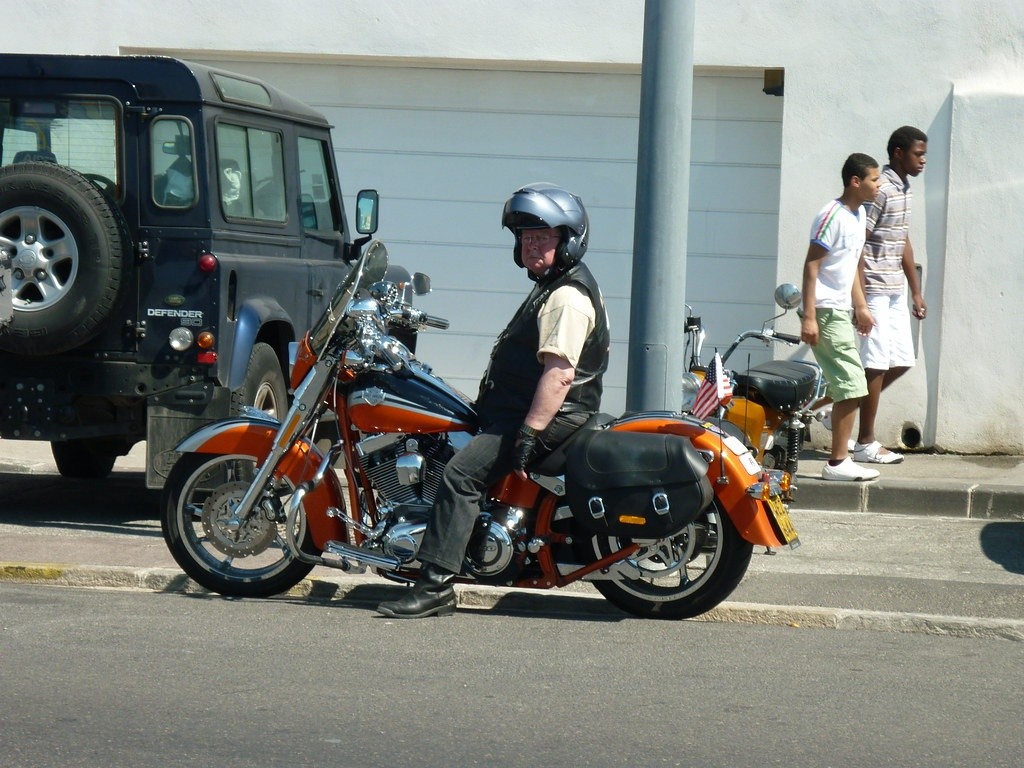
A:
[162,189,799,620]
[682,282,828,556]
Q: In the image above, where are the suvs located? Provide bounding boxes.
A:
[0,52,380,491]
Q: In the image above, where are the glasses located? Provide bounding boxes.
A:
[520,234,563,244]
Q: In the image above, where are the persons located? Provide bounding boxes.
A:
[375,188,608,618]
[852,125,928,465]
[801,151,881,479]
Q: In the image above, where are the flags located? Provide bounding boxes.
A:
[692,353,732,420]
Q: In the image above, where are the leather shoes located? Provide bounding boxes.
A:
[378,583,457,619]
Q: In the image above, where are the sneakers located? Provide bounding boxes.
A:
[822,456,880,481]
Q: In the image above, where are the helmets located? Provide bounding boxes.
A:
[501,188,590,271]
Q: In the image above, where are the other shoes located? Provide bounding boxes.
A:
[819,408,856,451]
[852,441,905,464]
